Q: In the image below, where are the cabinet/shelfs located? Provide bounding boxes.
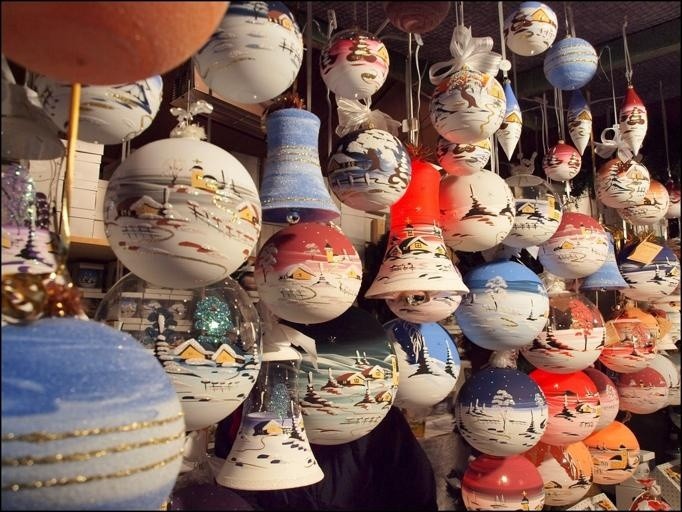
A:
[68,235,261,355]
